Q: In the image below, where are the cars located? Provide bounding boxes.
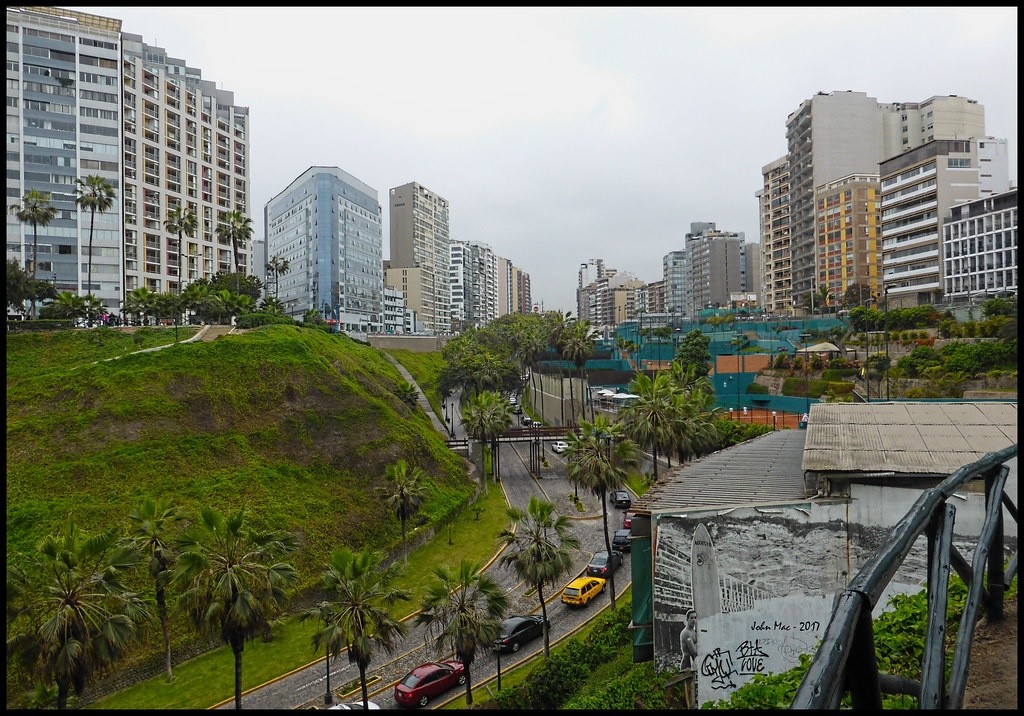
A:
[395,660,465,707]
[611,529,632,551]
[587,549,624,577]
[327,700,379,710]
[521,415,531,424]
[551,441,568,453]
[508,392,523,414]
[622,509,636,528]
[528,421,543,428]
[491,614,551,653]
[609,491,632,509]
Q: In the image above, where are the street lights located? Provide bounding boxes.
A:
[318,610,335,703]
[884,281,898,399]
[451,403,455,437]
[737,334,744,421]
[675,327,681,348]
[864,297,877,402]
[799,334,811,414]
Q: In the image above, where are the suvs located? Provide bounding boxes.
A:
[561,576,607,608]
